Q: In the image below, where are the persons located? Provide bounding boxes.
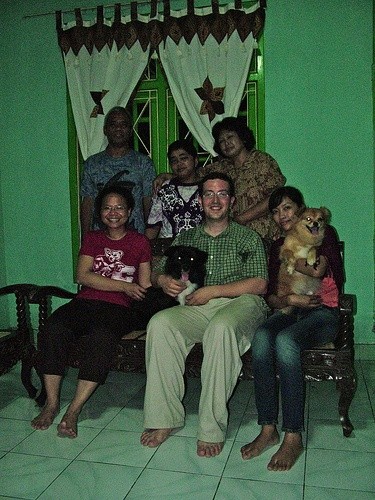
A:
[240,186,344,471]
[80,106,288,263]
[141,172,268,457]
[31,187,153,439]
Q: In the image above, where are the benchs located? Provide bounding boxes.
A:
[26,236,358,438]
[0,282,39,399]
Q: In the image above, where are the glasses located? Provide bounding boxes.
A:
[201,191,231,199]
[101,205,128,211]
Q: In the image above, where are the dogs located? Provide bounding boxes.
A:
[273,204,332,313]
[125,245,208,331]
